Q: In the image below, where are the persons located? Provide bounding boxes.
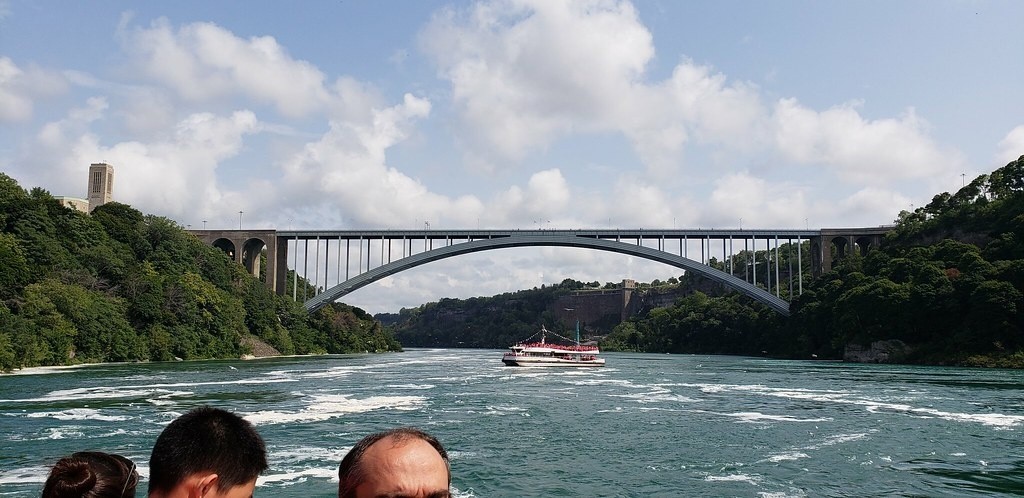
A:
[148,405,270,498]
[339,427,451,498]
[42,451,141,498]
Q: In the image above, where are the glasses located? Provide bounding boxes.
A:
[71,451,136,498]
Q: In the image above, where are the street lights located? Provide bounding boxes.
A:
[960,173,966,186]
[202,220,208,229]
[238,211,244,230]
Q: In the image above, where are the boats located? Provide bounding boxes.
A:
[502,323,606,368]
